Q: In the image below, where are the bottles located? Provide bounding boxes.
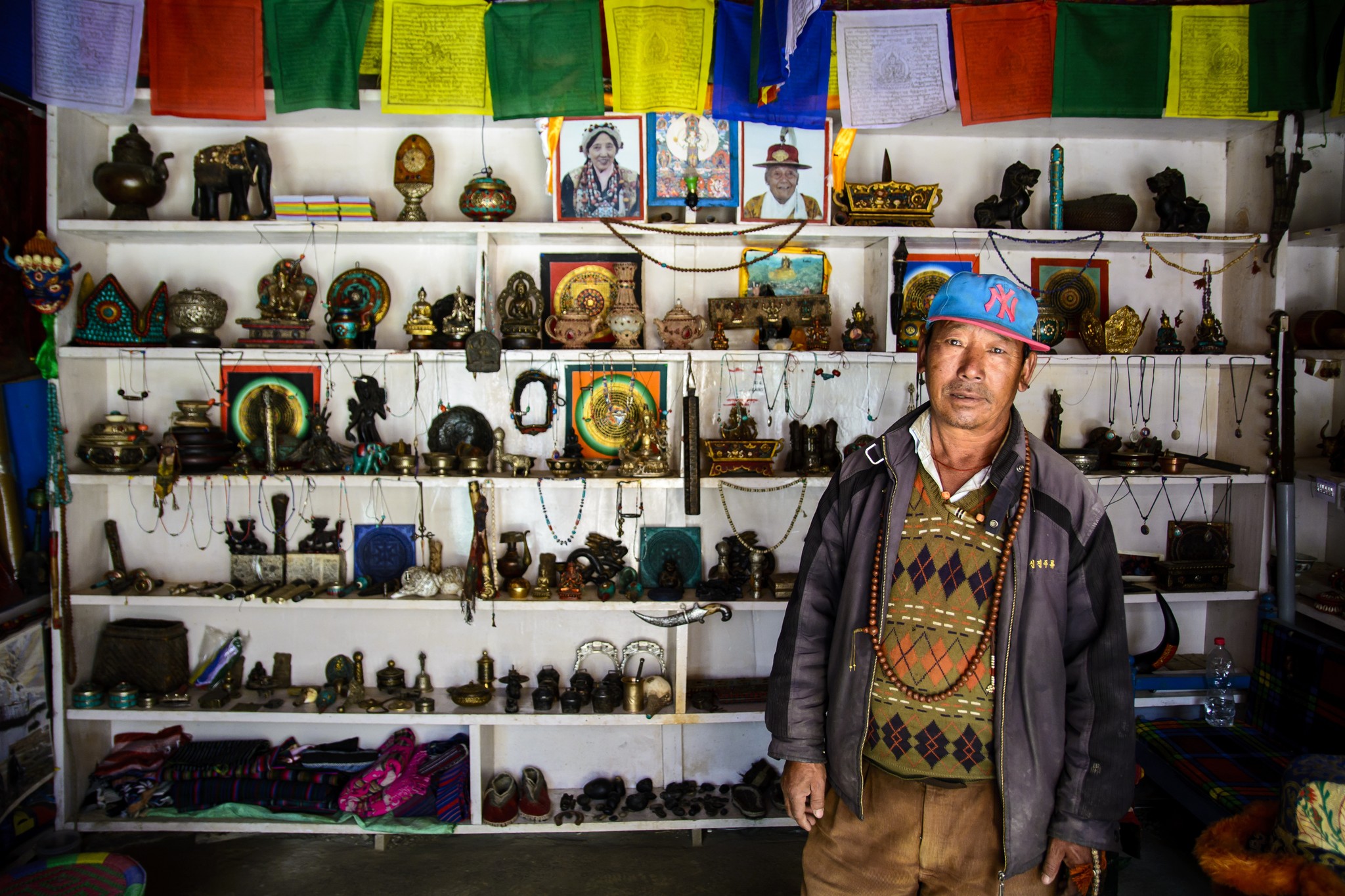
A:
[1204,637,1236,727]
[459,166,516,221]
[605,262,646,349]
[1026,295,1067,355]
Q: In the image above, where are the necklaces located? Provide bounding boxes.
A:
[121,477,648,562]
[719,479,811,555]
[952,230,1260,560]
[587,159,622,209]
[870,427,1035,704]
[48,382,73,507]
[115,347,924,451]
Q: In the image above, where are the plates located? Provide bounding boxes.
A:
[327,262,391,327]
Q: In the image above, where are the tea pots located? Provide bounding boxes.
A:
[653,298,708,350]
[545,299,605,349]
[93,122,174,220]
[324,299,372,349]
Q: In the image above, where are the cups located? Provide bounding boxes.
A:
[621,677,645,712]
[531,663,625,714]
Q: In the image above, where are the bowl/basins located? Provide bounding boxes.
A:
[1061,454,1099,474]
[1270,551,1317,573]
[446,681,496,708]
[1111,452,1158,475]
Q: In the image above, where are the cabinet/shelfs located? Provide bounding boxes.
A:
[45,88,1345,850]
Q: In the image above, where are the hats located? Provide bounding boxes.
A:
[753,128,813,169]
[581,122,621,158]
[924,271,1053,353]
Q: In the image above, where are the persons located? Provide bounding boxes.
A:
[268,272,296,318]
[561,122,641,217]
[745,127,823,221]
[407,287,432,324]
[764,271,1135,896]
[508,278,534,324]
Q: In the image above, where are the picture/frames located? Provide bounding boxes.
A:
[220,365,321,432]
[555,115,644,221]
[646,112,739,207]
[540,254,644,348]
[1030,258,1110,325]
[741,121,828,223]
[564,365,667,465]
